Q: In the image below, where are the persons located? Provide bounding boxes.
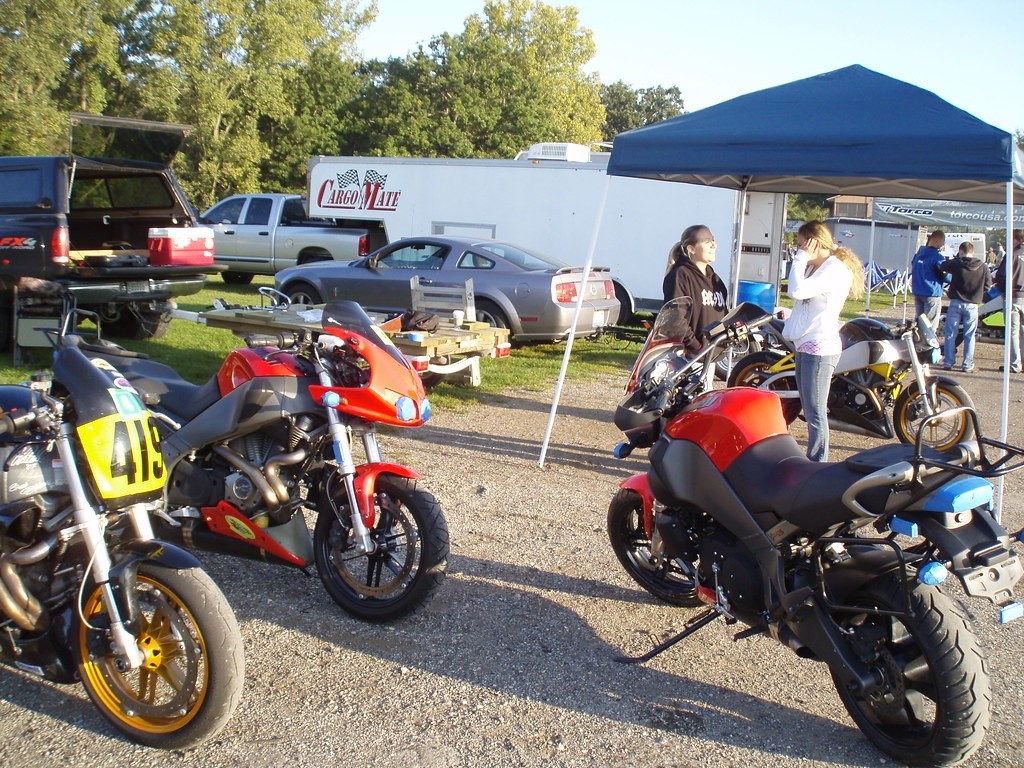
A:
[912,231,952,347]
[986,247,997,265]
[663,225,731,393]
[993,229,1024,374]
[781,219,864,463]
[997,243,1006,265]
[941,242,993,373]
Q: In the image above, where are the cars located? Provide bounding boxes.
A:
[269,234,622,348]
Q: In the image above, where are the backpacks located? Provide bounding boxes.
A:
[381,310,440,333]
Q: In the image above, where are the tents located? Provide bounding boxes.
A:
[535,61,1024,531]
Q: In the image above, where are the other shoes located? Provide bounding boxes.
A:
[963,368,970,371]
[944,366,952,370]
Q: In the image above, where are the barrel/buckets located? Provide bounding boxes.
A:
[738,280,775,315]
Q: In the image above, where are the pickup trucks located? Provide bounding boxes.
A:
[196,192,371,283]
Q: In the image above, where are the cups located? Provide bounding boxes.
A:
[453,310,465,328]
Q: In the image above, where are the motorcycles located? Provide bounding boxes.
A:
[0,341,245,751]
[604,294,1023,767]
[31,296,451,624]
[714,301,976,452]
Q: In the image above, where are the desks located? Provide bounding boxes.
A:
[197,304,514,387]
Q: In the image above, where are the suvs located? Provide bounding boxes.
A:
[0,113,231,352]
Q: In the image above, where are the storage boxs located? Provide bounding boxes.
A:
[148,225,215,266]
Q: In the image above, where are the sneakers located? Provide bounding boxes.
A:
[999,365,1017,373]
